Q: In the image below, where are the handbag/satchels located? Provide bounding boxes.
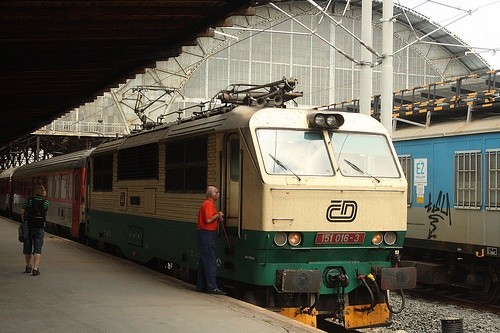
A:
[19,223,28,242]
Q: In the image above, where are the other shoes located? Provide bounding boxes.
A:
[210,288,225,294]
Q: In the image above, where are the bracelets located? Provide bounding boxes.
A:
[217,212,221,219]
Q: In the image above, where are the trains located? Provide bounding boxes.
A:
[0,75,418,331]
[307,68,500,311]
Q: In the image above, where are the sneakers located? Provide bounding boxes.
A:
[25,265,32,273]
[32,267,40,276]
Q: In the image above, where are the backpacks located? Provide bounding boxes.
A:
[27,198,46,228]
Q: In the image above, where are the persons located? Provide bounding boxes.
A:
[197,184,225,295]
[22,185,49,276]
